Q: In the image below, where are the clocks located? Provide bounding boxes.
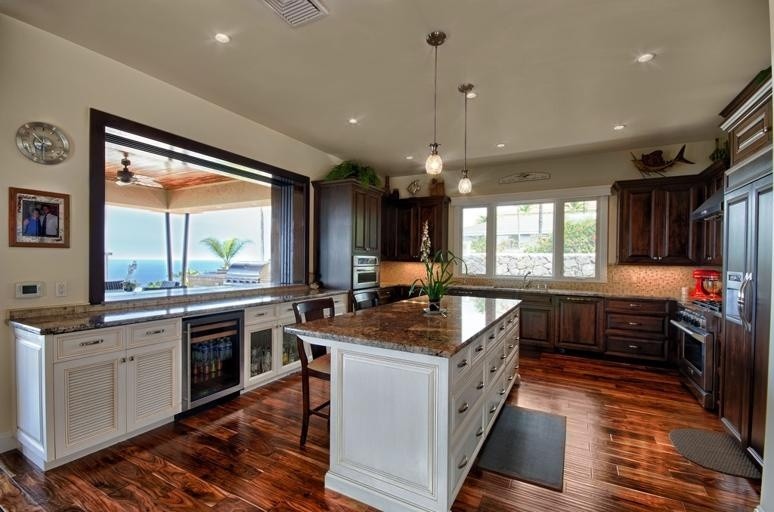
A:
[14,121,72,166]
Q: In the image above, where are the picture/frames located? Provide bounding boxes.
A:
[8,186,71,248]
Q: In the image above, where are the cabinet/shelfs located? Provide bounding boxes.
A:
[311,178,385,256]
[555,294,604,358]
[11,317,183,474]
[513,292,556,354]
[391,196,451,263]
[239,299,311,395]
[608,174,701,266]
[604,297,678,368]
[720,173,770,470]
[702,158,723,266]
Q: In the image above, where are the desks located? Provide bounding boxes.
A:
[283,293,525,512]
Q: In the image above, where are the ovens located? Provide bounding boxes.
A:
[351,288,381,312]
[670,319,715,411]
[353,255,381,290]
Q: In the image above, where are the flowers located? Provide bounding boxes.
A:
[407,220,466,301]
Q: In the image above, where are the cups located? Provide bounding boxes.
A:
[681,286,689,303]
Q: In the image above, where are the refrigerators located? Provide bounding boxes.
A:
[177,309,247,413]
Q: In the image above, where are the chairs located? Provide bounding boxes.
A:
[291,296,335,447]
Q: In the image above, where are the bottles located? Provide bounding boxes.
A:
[193,337,232,376]
[383,175,391,198]
[429,178,438,196]
[391,189,399,200]
[436,173,445,197]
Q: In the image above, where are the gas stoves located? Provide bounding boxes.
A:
[693,298,724,313]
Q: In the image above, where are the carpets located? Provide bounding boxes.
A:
[476,404,567,493]
[669,428,761,481]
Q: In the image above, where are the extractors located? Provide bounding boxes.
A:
[689,183,724,225]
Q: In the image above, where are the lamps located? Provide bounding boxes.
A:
[457,83,474,195]
[425,30,447,176]
[116,150,134,183]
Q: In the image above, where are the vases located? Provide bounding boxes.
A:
[423,297,446,314]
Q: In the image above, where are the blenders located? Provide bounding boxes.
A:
[690,268,722,302]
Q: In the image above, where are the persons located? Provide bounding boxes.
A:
[39,205,59,236]
[22,207,42,237]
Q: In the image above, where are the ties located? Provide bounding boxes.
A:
[41,215,47,236]
